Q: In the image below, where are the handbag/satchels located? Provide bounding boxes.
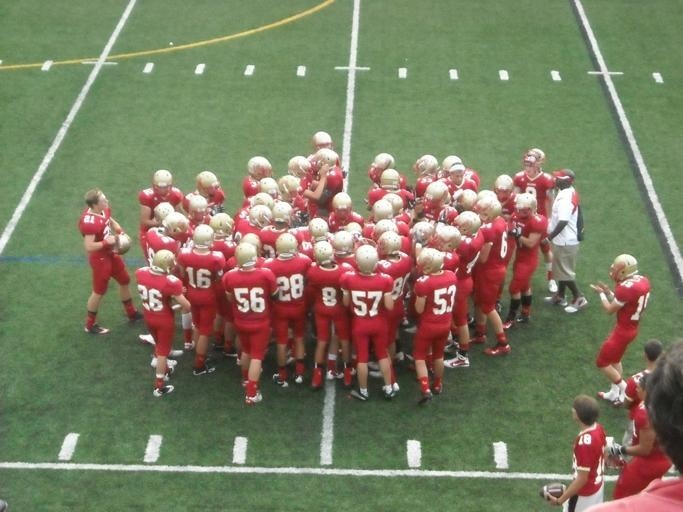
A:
[576,202,585,241]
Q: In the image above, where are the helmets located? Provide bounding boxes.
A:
[249,205,272,223]
[454,211,481,235]
[287,156,311,175]
[240,231,262,250]
[373,153,395,169]
[247,157,272,178]
[379,169,399,189]
[523,148,545,166]
[251,194,274,210]
[278,175,302,197]
[152,249,175,271]
[153,204,174,222]
[608,254,638,279]
[416,154,438,174]
[196,171,219,195]
[166,213,188,235]
[457,189,477,207]
[275,233,297,253]
[414,222,433,240]
[272,202,292,222]
[372,199,393,221]
[424,181,448,204]
[187,196,207,216]
[476,190,497,201]
[442,156,463,169]
[494,175,514,191]
[234,243,256,266]
[315,149,337,167]
[153,170,174,191]
[373,220,398,238]
[259,179,279,196]
[313,241,333,265]
[193,224,214,246]
[115,232,132,255]
[378,232,400,255]
[478,198,501,218]
[383,192,403,214]
[332,192,351,212]
[312,131,331,148]
[438,226,461,250]
[416,248,445,273]
[355,245,378,273]
[308,218,329,239]
[346,221,363,236]
[210,212,232,234]
[333,229,355,255]
[513,192,536,211]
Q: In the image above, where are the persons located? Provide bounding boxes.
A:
[139,170,183,257]
[262,234,314,388]
[458,197,512,356]
[370,231,411,379]
[178,224,226,376]
[547,395,603,512]
[605,375,672,500]
[306,241,354,390]
[494,174,517,313]
[325,232,359,381]
[236,232,266,374]
[542,168,587,313]
[409,248,458,407]
[443,211,481,368]
[515,148,560,294]
[580,340,683,512]
[134,250,192,397]
[624,340,662,445]
[80,188,145,335]
[341,244,396,402]
[590,254,652,406]
[209,212,235,353]
[223,243,280,405]
[504,193,546,332]
[147,212,196,357]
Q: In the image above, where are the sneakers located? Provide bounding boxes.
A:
[614,396,628,407]
[273,373,288,388]
[152,384,176,398]
[519,315,529,324]
[444,354,469,368]
[151,357,178,368]
[213,342,224,349]
[138,332,155,344]
[468,335,487,344]
[336,367,356,379]
[445,342,469,353]
[547,279,558,293]
[565,297,588,314]
[184,340,195,349]
[83,323,109,335]
[368,362,391,378]
[350,389,367,402]
[484,344,512,355]
[499,320,514,329]
[194,364,215,375]
[544,294,568,309]
[295,375,305,384]
[327,371,344,380]
[128,310,144,321]
[222,348,237,356]
[169,349,183,356]
[393,350,405,363]
[244,392,262,402]
[381,387,395,399]
[598,390,618,402]
[417,392,434,407]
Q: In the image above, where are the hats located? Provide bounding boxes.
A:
[449,165,465,172]
[553,168,575,180]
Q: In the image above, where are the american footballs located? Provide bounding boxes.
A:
[538,483,568,501]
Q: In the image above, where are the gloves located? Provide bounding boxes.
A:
[607,439,625,457]
